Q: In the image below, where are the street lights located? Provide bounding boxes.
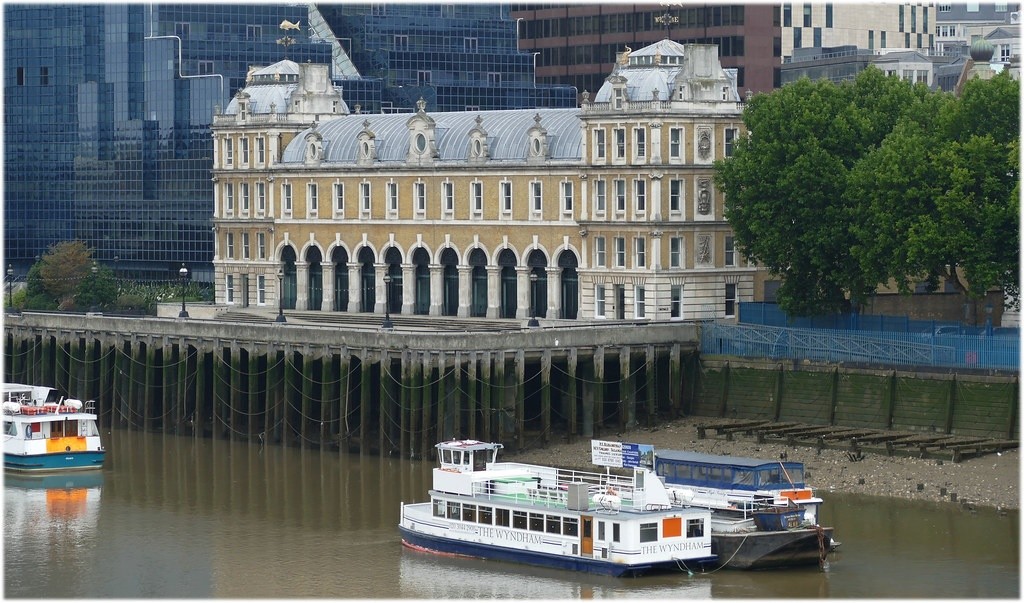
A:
[91,261,98,312]
[178,263,189,317]
[7,264,14,307]
[381,272,394,328]
[275,270,287,321]
[528,270,540,327]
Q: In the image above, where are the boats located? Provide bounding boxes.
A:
[396,438,719,576]
[2,471,101,541]
[394,546,713,599]
[3,382,107,477]
[607,448,841,572]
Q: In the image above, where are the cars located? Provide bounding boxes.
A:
[978,326,1018,338]
[915,324,966,347]
